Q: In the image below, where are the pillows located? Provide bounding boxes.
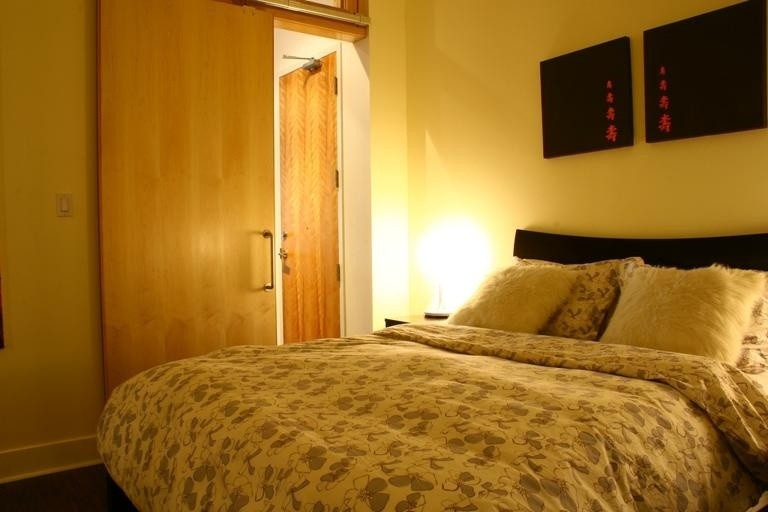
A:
[500,257,646,342]
[451,265,579,334]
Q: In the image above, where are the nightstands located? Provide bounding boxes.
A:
[385,316,445,328]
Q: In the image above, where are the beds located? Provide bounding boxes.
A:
[98,228,767,511]
